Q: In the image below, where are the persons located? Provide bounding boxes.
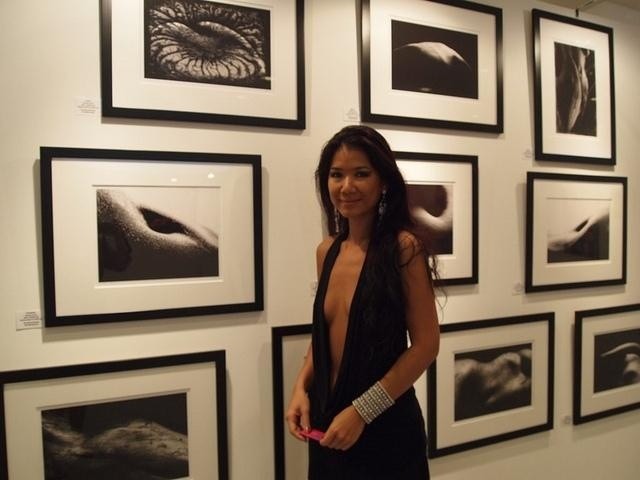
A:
[286,125,440,480]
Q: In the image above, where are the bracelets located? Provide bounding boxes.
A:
[352,381,395,424]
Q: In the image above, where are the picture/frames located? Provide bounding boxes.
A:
[4,350,236,479]
[395,151,479,289]
[527,172,628,295]
[355,0,502,133]
[426,311,558,462]
[573,301,640,424]
[39,154,267,330]
[97,2,306,131]
[530,9,617,165]
[271,322,311,479]
